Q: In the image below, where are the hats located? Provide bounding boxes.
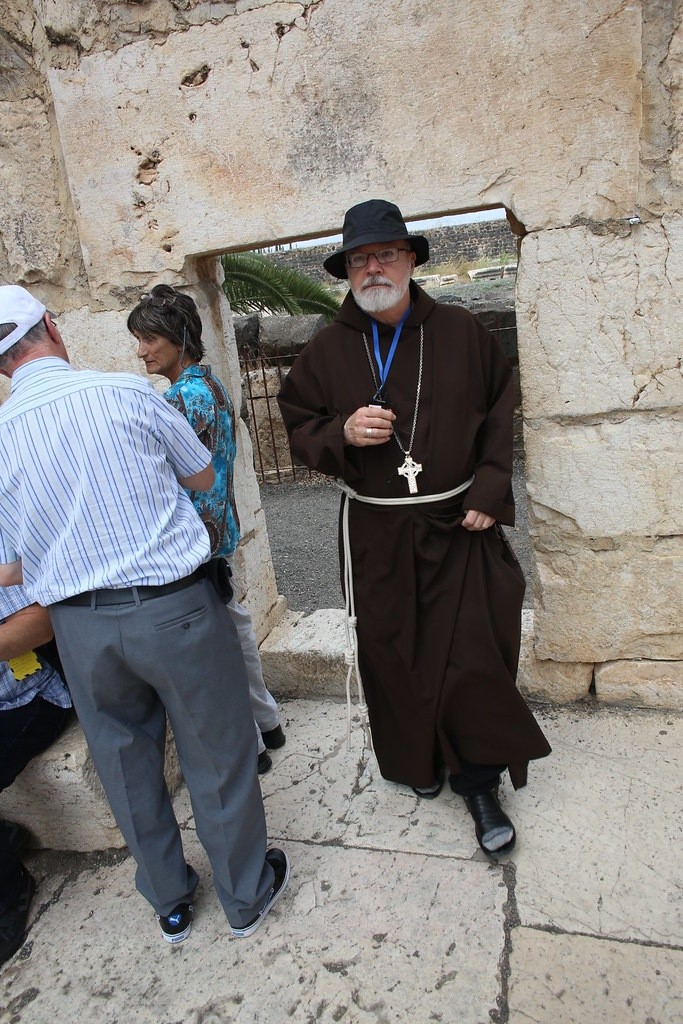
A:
[324,200,430,279]
[1,284,58,355]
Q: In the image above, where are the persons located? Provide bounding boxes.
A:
[0,285,290,960]
[275,199,552,858]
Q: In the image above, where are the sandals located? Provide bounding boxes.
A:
[410,778,445,800]
[463,780,518,857]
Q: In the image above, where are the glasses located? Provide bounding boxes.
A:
[138,292,172,307]
[343,246,410,269]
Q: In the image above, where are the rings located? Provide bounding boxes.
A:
[367,428,372,438]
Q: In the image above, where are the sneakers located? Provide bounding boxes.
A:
[156,899,193,944]
[0,862,35,965]
[231,848,290,938]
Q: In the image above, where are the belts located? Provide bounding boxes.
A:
[46,564,209,606]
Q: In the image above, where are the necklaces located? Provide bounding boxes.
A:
[362,323,424,493]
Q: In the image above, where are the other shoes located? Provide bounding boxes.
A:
[257,751,272,775]
[262,724,285,750]
